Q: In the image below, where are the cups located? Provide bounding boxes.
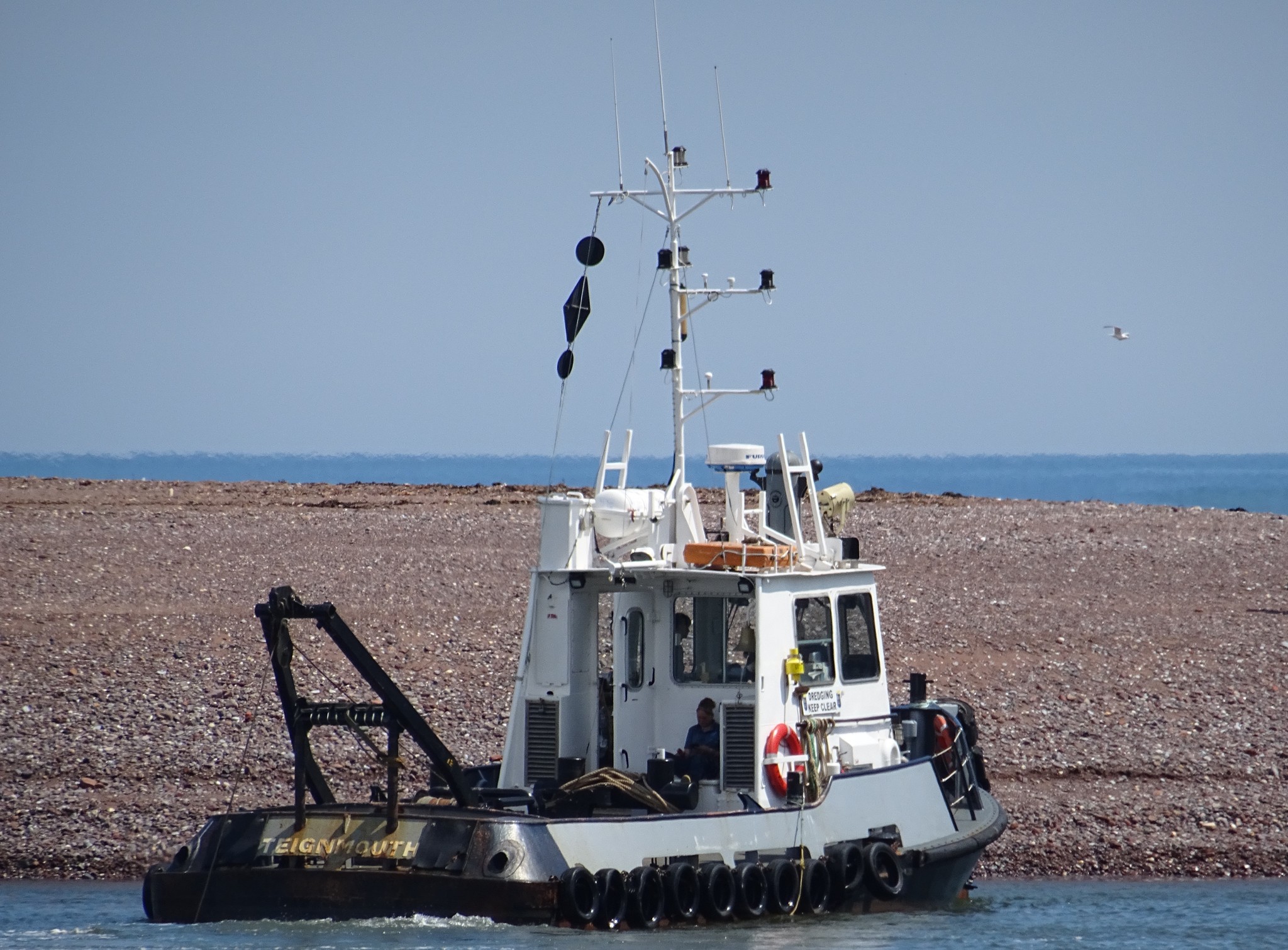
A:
[657,748,665,760]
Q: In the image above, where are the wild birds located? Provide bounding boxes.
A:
[1104,326,1130,340]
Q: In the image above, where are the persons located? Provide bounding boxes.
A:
[673,614,690,682]
[674,698,720,787]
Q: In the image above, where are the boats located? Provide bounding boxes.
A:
[141,14,1010,932]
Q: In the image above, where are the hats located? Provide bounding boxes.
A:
[696,698,716,715]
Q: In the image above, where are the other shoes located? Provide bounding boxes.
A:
[681,774,691,784]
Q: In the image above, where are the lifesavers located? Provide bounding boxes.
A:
[934,714,955,780]
[559,841,904,930]
[765,723,805,796]
